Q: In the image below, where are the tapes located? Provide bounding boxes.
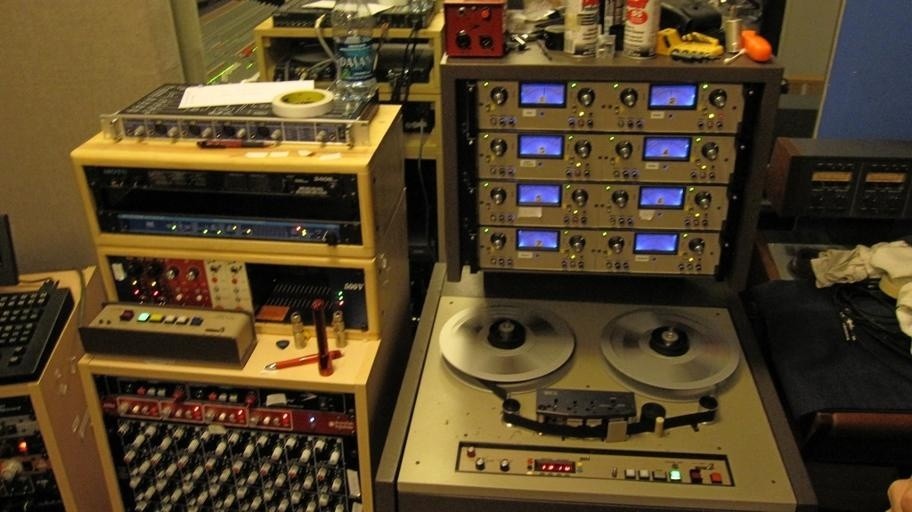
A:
[271,89,334,118]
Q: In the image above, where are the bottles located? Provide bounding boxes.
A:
[332,310,347,348]
[289,311,305,349]
[328,0,378,105]
[725,19,743,54]
[563,1,601,62]
[622,0,661,62]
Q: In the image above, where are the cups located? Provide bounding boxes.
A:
[609,23,624,52]
[544,25,565,51]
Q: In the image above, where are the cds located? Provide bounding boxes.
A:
[601,307,742,391]
[437,303,576,383]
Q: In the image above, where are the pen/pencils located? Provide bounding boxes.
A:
[265,350,342,370]
[537,40,552,60]
[197,141,274,148]
